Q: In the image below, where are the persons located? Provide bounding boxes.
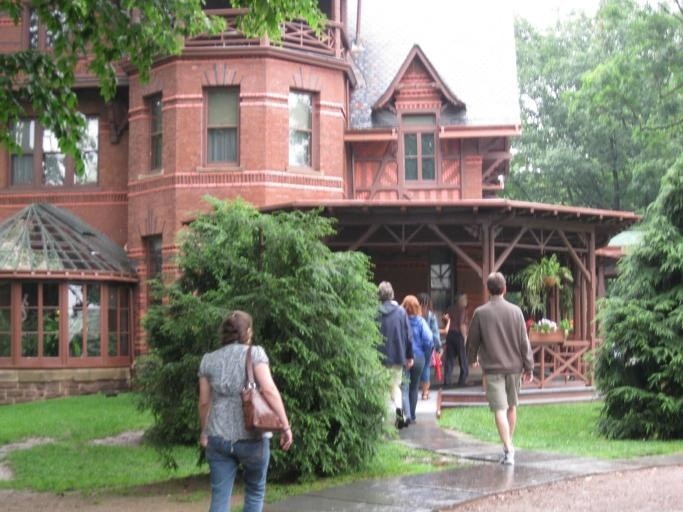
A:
[398,294,435,427]
[464,271,534,465]
[429,312,452,369]
[193,309,294,512]
[519,305,534,333]
[375,281,415,429]
[414,291,443,401]
[529,307,557,332]
[438,290,474,387]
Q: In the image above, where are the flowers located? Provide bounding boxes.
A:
[531,319,557,334]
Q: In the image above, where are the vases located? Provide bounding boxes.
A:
[527,328,565,343]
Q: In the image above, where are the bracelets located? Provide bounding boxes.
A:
[281,425,291,434]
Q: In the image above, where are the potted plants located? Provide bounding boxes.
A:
[541,253,560,287]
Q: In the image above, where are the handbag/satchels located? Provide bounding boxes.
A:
[240,385,283,432]
[430,349,442,367]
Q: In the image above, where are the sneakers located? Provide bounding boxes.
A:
[397,408,416,429]
[500,448,515,466]
